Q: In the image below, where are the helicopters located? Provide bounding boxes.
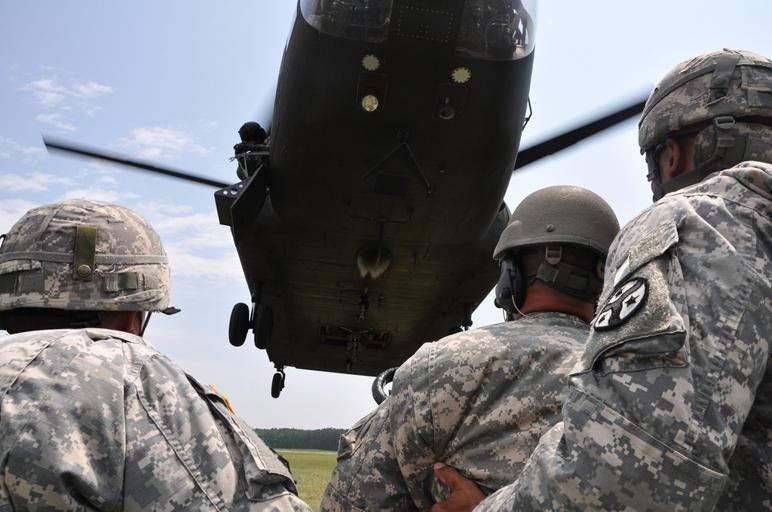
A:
[42,0,653,407]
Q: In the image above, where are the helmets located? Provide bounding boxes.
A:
[636,48,772,152]
[0,199,171,315]
[491,183,620,263]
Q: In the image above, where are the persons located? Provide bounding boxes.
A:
[0,199,313,511]
[319,186,621,512]
[428,49,772,512]
